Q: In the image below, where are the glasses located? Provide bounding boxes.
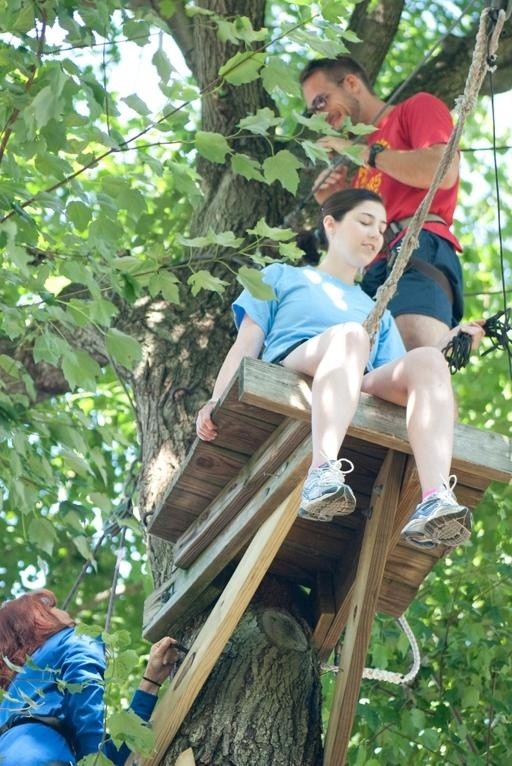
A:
[300,78,348,121]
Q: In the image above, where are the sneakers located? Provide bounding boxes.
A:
[296,457,358,524]
[398,487,473,550]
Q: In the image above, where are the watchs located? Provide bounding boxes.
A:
[368,142,384,167]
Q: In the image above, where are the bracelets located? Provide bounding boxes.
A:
[142,677,161,688]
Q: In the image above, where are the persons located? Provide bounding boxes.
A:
[0,590,179,766]
[192,186,487,551]
[299,54,466,354]
